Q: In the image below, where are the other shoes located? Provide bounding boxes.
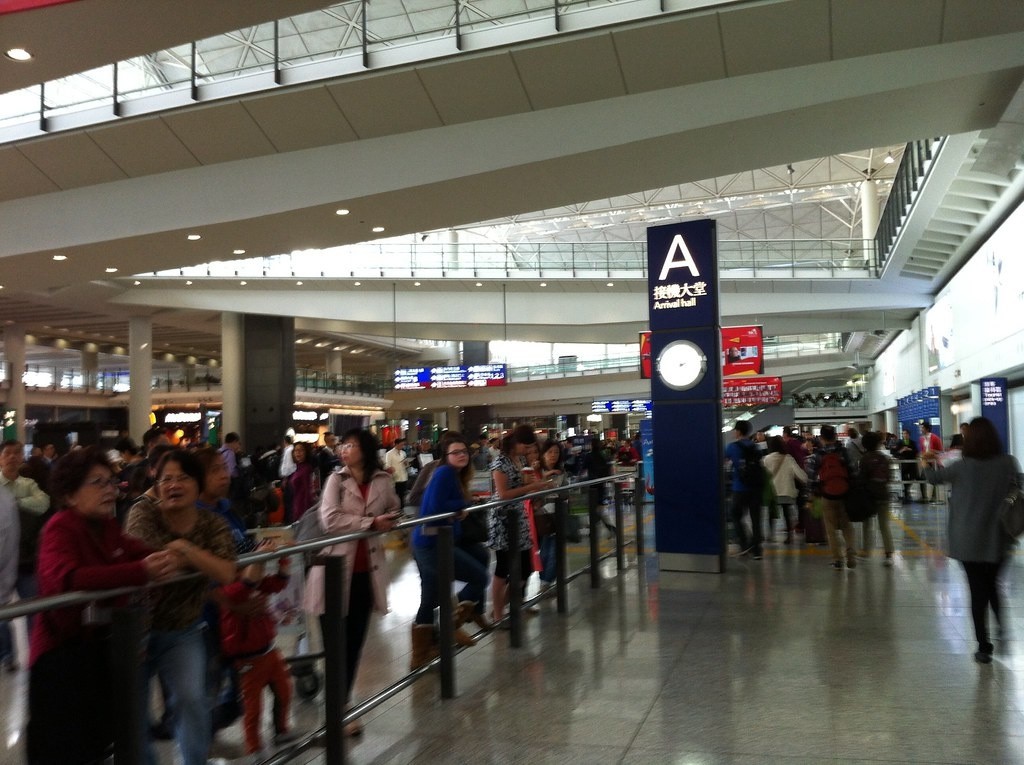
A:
[834,561,844,568]
[340,705,362,737]
[2,656,16,671]
[519,598,540,614]
[975,651,993,664]
[273,727,305,744]
[847,557,857,568]
[884,558,894,566]
[751,547,763,560]
[474,612,494,628]
[740,545,752,554]
[766,536,777,542]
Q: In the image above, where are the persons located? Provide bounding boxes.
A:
[727,346,741,362]
[918,416,1024,657]
[0,415,646,764]
[726,420,969,571]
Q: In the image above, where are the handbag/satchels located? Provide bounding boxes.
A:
[562,501,581,543]
[995,484,1024,536]
[295,474,344,543]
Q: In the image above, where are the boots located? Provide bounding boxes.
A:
[453,600,477,647]
[410,622,441,671]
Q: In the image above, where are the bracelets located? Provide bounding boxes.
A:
[180,541,195,554]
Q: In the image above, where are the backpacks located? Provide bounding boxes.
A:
[862,454,889,485]
[733,441,765,489]
[815,447,849,496]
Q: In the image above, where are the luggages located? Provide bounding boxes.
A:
[803,488,827,543]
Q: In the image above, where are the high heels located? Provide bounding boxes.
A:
[784,535,794,544]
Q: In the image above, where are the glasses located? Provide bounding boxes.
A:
[86,476,123,487]
[445,448,469,455]
[338,442,362,450]
